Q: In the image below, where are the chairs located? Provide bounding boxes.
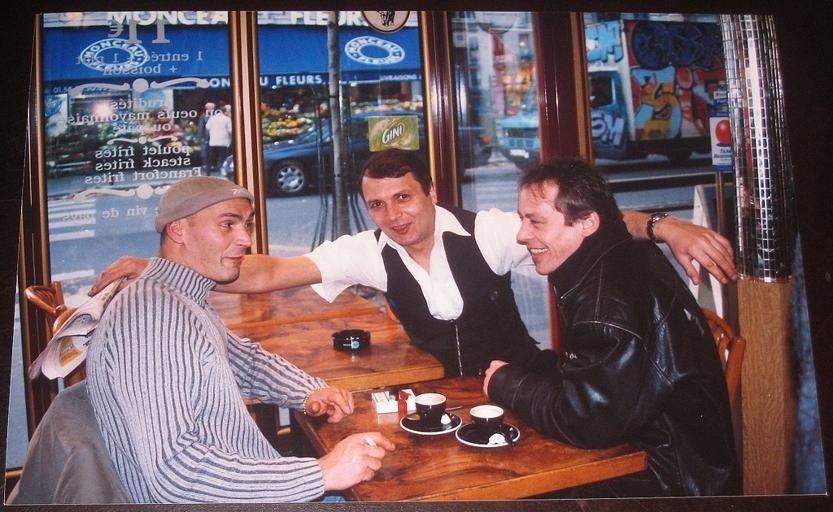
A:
[508,256,563,355]
[701,307,746,418]
[21,281,81,406]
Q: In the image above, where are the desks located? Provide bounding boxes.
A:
[240,312,445,420]
[209,286,378,330]
[287,375,649,499]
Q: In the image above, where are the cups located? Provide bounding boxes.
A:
[470,405,505,434]
[414,392,446,425]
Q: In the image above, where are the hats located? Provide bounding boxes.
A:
[155,176,254,234]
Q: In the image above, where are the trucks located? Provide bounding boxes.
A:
[492,19,727,173]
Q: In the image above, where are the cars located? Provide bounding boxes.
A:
[219,110,493,198]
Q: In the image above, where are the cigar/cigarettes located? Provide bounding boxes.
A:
[363,436,377,447]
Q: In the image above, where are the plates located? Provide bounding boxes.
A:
[400,412,463,436]
[455,421,520,449]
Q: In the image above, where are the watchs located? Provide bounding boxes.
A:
[646,212,671,244]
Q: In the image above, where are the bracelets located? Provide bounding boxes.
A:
[300,387,328,416]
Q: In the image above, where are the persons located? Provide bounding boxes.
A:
[84,147,742,380]
[481,157,743,495]
[83,175,395,505]
[257,83,412,123]
[139,100,234,176]
